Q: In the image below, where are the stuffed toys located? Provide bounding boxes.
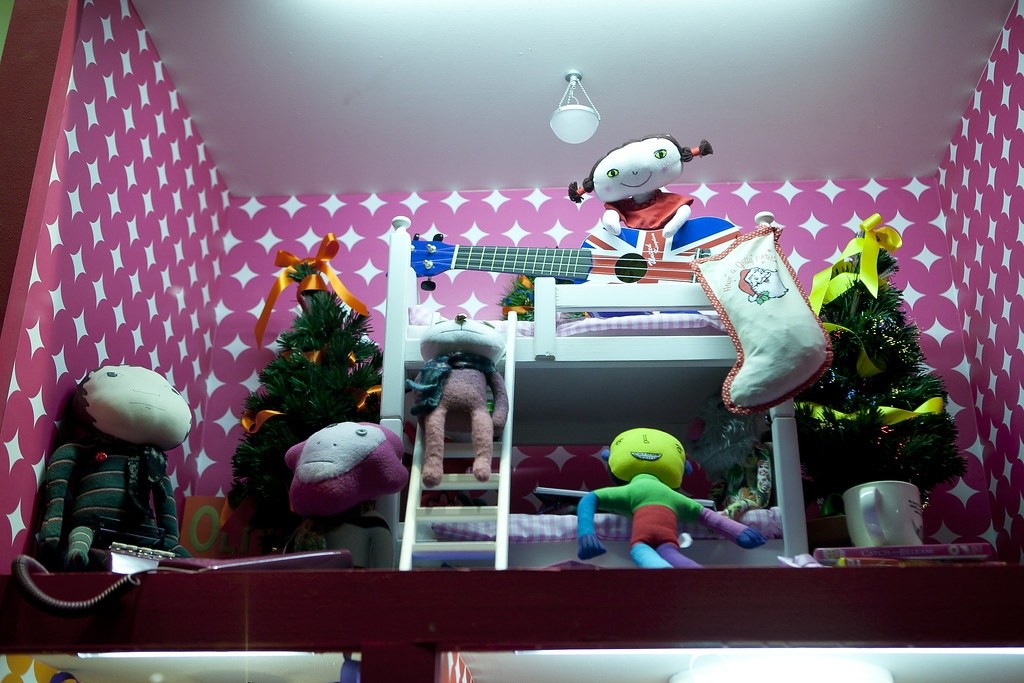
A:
[565,133,714,239]
[282,420,407,515]
[34,364,194,567]
[577,427,769,569]
[403,309,508,485]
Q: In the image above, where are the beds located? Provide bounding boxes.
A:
[370,210,809,572]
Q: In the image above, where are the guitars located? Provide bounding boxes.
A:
[410,217,737,322]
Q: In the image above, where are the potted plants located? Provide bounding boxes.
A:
[789,237,970,551]
[231,279,388,565]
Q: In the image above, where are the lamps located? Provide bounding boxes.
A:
[549,69,601,144]
